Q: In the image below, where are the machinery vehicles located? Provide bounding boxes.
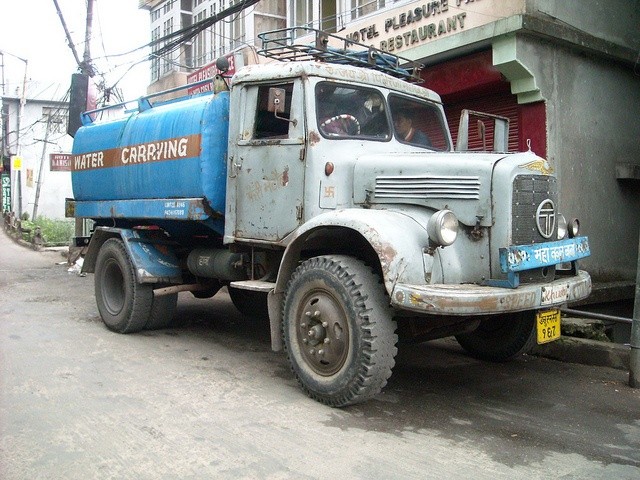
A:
[71,27,593,407]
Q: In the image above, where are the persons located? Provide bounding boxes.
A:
[392,108,432,147]
[320,98,358,135]
